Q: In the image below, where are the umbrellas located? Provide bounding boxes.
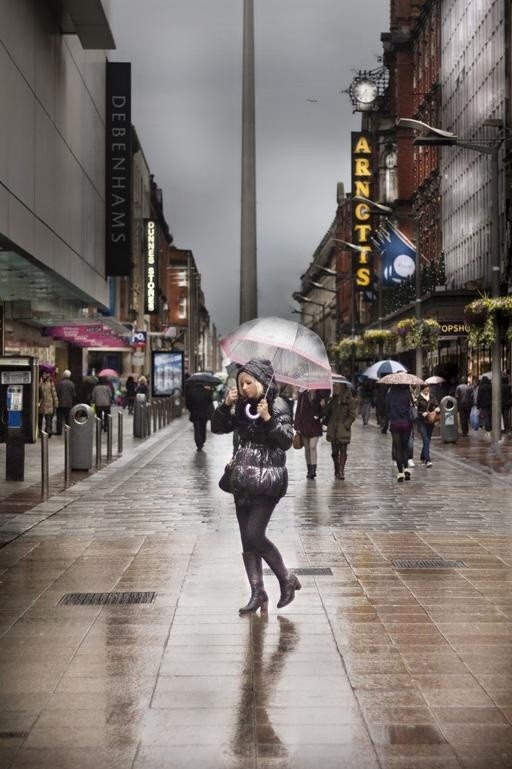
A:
[218,316,333,420]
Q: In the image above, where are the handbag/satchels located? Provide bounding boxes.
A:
[409,404,418,421]
[219,462,236,493]
[426,409,442,424]
[293,431,304,449]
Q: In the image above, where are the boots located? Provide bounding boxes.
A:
[239,553,268,613]
[264,546,301,608]
[332,455,347,479]
[306,464,316,479]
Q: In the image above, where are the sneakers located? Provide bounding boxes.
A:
[397,473,404,482]
[404,468,411,480]
[408,459,415,467]
[426,461,432,468]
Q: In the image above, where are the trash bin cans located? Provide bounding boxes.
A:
[134,394,147,437]
[440,395,458,444]
[68,403,94,473]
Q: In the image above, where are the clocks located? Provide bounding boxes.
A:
[341,66,387,114]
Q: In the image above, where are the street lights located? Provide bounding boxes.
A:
[350,194,425,396]
[289,237,385,390]
[394,114,504,454]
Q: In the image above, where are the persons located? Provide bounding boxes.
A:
[211,358,303,614]
[219,361,244,458]
[39,363,149,437]
[277,376,511,482]
[186,380,217,451]
